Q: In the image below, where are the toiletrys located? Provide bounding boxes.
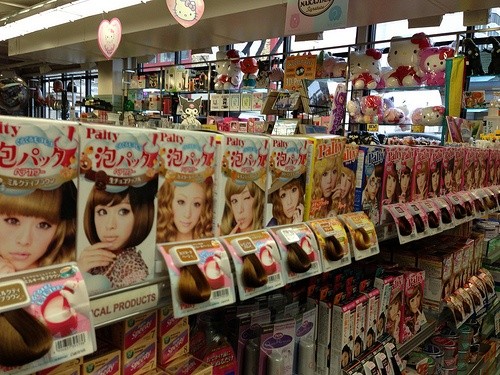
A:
[0,114,500,375]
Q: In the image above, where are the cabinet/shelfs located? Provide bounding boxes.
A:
[0,27,499,374]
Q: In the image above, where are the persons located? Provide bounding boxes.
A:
[0,146,500,372]
